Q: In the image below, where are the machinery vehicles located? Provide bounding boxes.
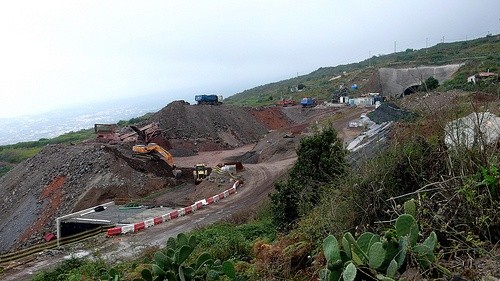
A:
[132,143,175,169]
[301,97,317,107]
[192,163,208,184]
[94,124,121,144]
[276,97,296,107]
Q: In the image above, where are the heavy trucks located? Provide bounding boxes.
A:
[194,95,223,105]
